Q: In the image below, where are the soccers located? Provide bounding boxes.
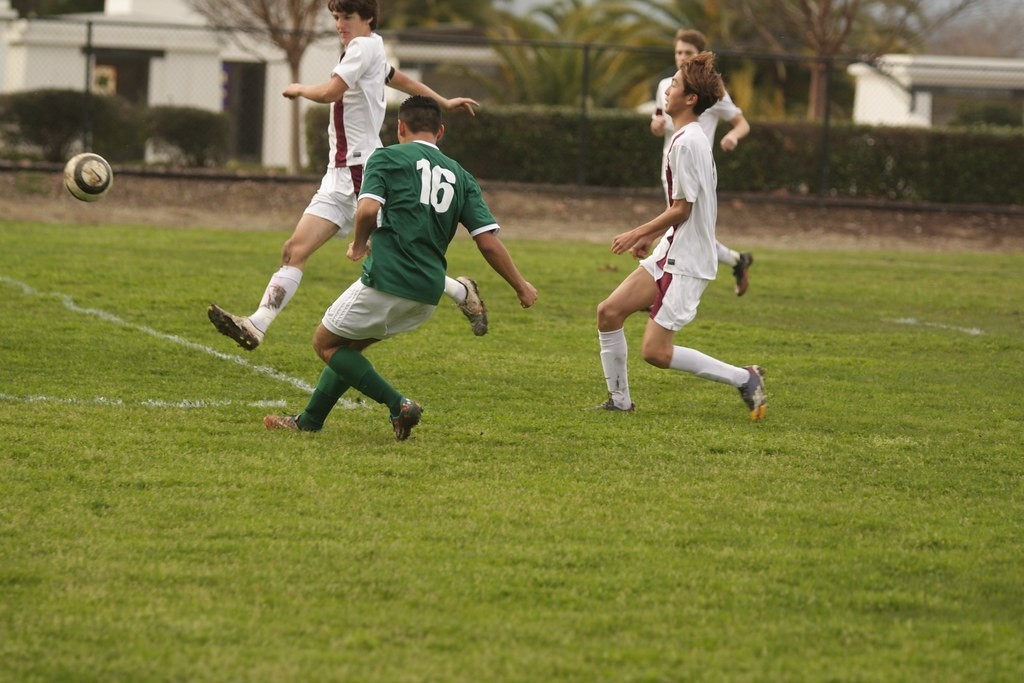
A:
[65,154,115,203]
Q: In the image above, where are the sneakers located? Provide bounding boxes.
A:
[264,412,301,432]
[589,392,636,410]
[207,305,263,350]
[454,277,487,337]
[733,251,753,296]
[738,366,765,423]
[389,396,424,441]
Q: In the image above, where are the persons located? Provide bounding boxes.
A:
[595,51,767,423]
[208,0,487,351]
[262,95,538,443]
[650,28,754,296]
